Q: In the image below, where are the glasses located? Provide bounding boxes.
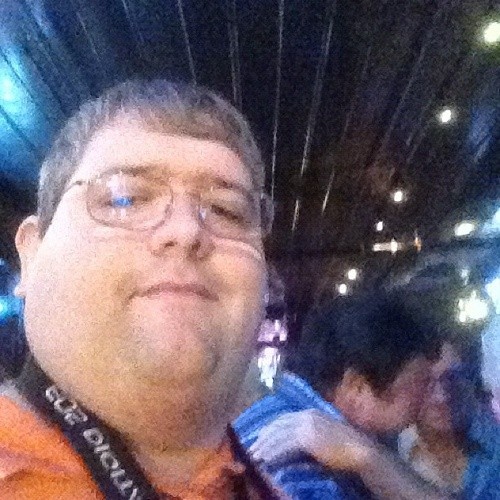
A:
[60,170,274,241]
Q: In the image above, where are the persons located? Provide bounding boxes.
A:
[0,78,289,500]
[230,295,500,500]
